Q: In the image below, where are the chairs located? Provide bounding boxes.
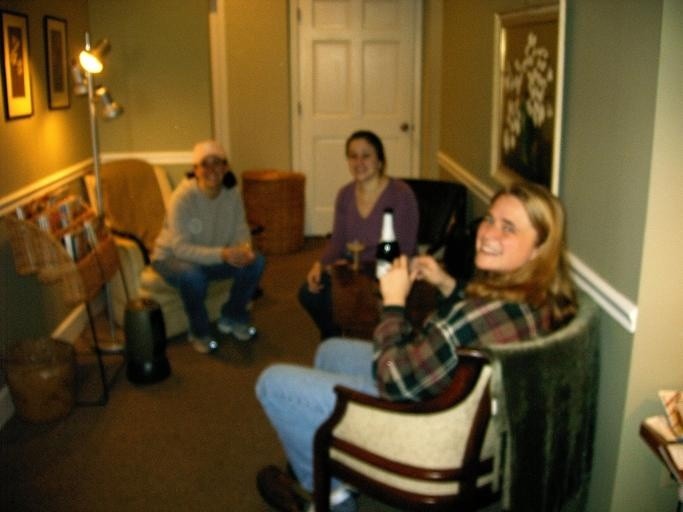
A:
[401,178,467,266]
[310,283,600,510]
[84,158,253,340]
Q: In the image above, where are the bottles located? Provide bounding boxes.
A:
[375,207,400,282]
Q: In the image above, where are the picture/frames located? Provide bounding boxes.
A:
[0,9,34,121]
[43,15,70,110]
[488,1,566,199]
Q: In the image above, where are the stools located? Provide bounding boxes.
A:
[2,341,75,421]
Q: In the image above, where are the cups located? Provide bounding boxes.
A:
[346,242,364,272]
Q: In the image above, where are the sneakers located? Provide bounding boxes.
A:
[187,332,213,353]
[217,317,252,342]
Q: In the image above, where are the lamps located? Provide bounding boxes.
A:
[68,31,126,354]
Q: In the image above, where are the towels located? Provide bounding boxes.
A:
[486,287,599,510]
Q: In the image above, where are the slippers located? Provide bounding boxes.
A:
[256,462,311,511]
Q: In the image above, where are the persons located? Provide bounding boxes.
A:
[151,141,266,353]
[254,182,577,511]
[298,130,420,340]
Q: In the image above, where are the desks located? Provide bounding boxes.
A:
[333,260,443,339]
[241,171,306,255]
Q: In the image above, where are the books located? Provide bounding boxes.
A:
[17,184,106,263]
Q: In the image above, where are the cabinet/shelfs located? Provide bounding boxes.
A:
[0,185,133,407]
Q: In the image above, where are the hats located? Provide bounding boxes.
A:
[191,140,227,166]
